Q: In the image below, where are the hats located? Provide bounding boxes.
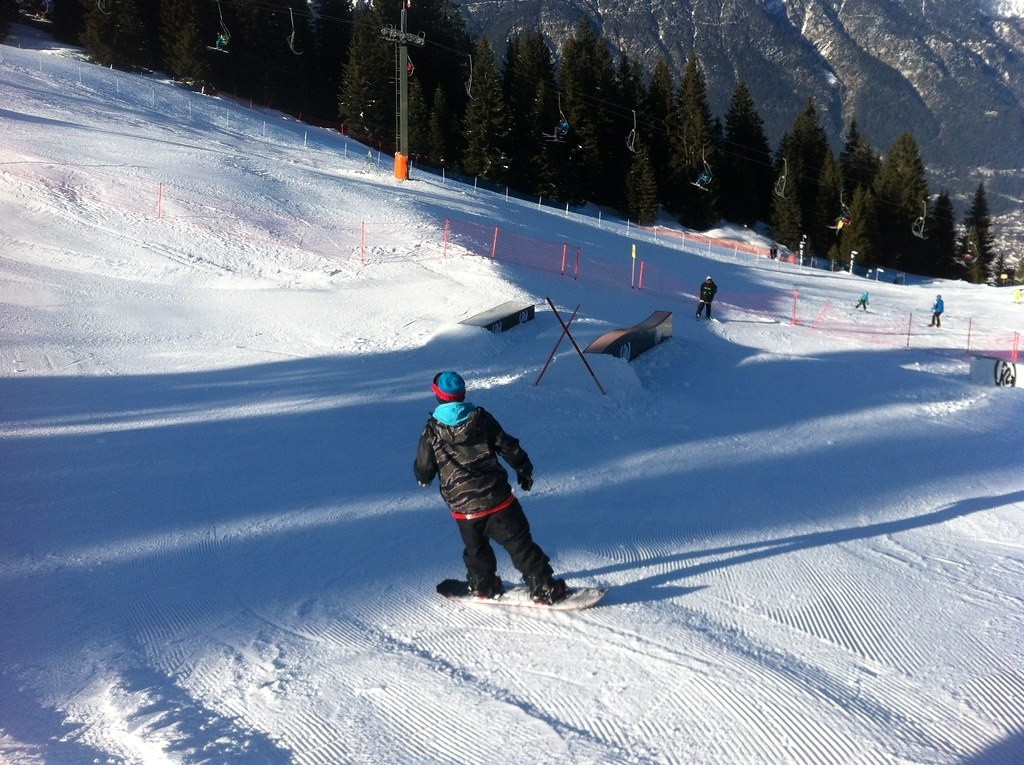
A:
[706,276,712,280]
[436,371,465,403]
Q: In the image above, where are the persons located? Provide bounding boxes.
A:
[835,213,852,229]
[696,173,710,187]
[1014,288,1024,302]
[553,119,567,141]
[769,245,778,260]
[928,295,943,327]
[695,276,718,322]
[394,62,413,80]
[959,250,974,265]
[855,291,869,312]
[216,30,229,49]
[414,371,572,605]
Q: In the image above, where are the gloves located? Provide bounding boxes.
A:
[517,463,533,491]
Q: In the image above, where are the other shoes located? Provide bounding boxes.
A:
[534,577,567,605]
[705,314,712,320]
[478,575,502,599]
[696,313,700,320]
[928,323,935,326]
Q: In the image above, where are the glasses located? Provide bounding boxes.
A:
[433,372,446,384]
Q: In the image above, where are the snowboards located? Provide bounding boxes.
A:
[436,575,610,610]
